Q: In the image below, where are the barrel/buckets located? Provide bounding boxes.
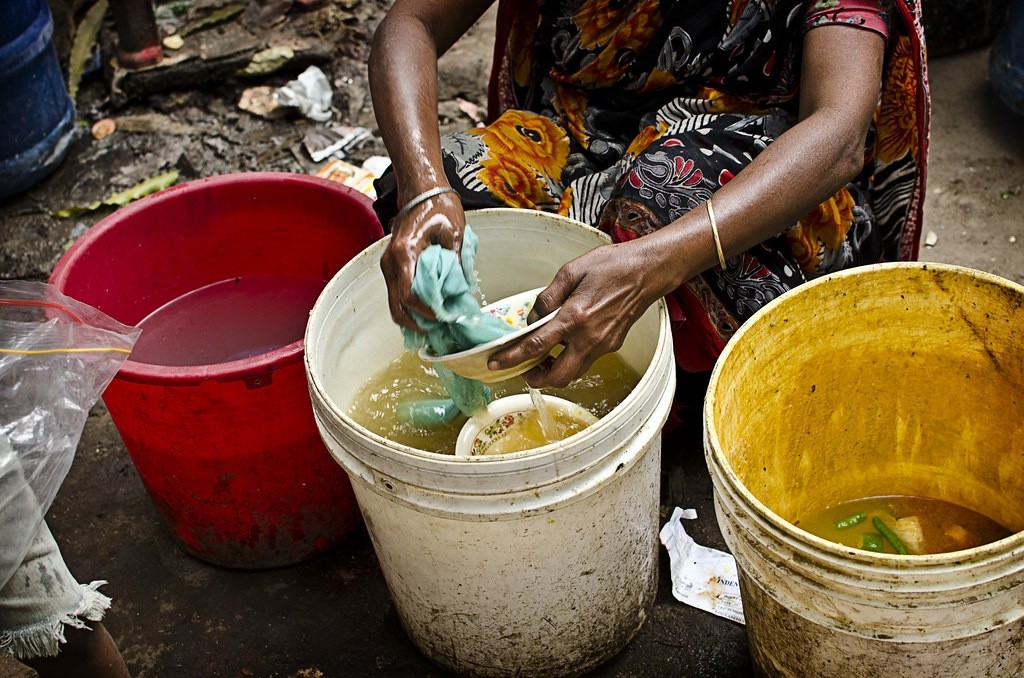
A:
[41,163,387,576]
[2,2,80,183]
[301,197,680,676]
[703,257,1023,675]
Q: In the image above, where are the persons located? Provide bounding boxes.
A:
[365,1,933,442]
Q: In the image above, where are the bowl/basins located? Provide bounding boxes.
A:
[414,287,564,385]
[456,390,597,458]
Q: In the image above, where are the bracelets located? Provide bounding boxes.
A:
[388,185,459,230]
[703,198,731,273]
